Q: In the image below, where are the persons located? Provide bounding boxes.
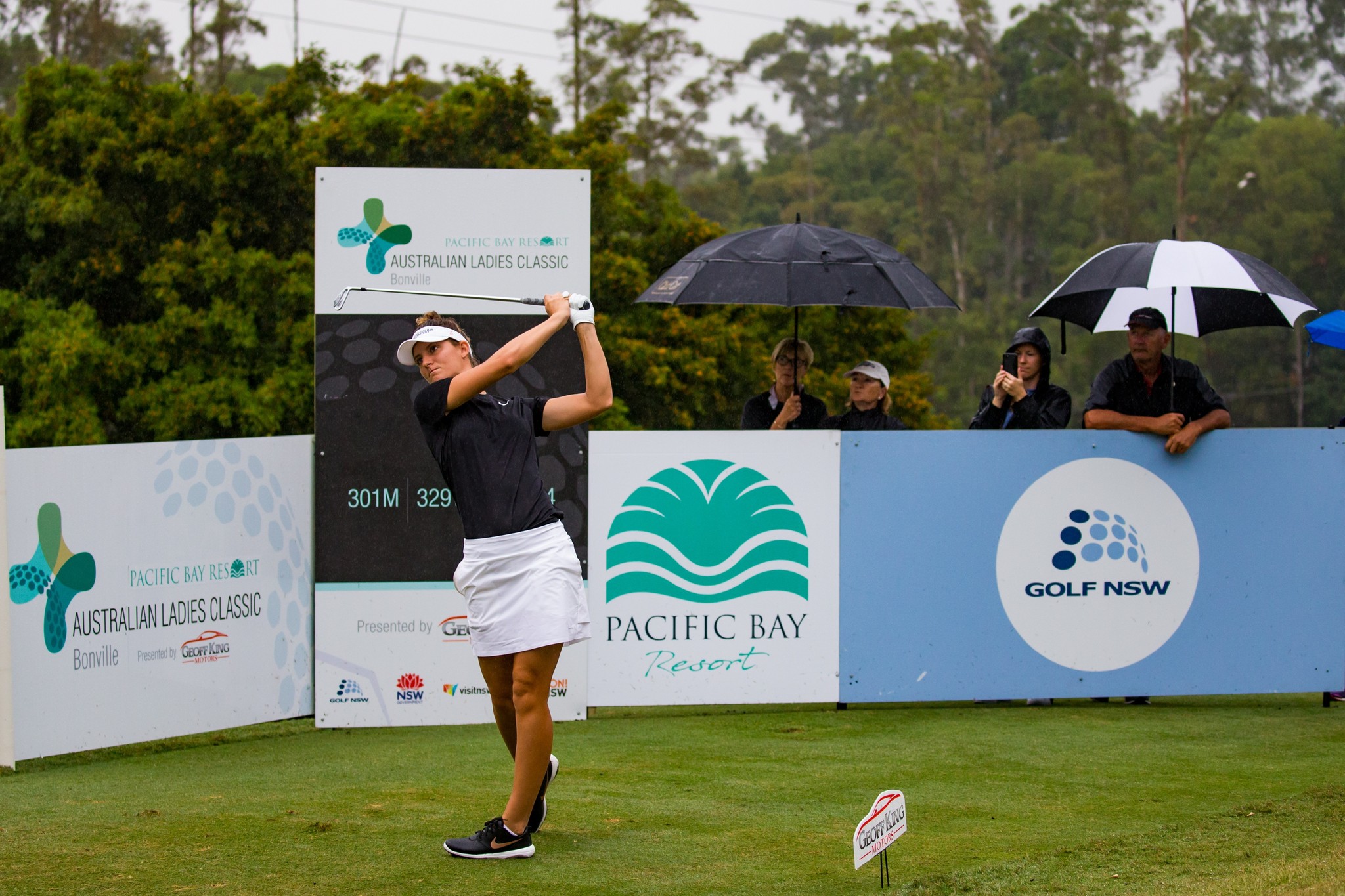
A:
[396,288,615,860]
[740,338,829,429]
[1082,308,1231,455]
[827,360,910,430]
[968,326,1072,430]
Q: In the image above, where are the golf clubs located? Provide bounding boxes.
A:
[332,286,591,312]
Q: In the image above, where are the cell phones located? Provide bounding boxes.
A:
[1002,353,1018,378]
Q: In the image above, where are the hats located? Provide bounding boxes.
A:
[1124,307,1167,332]
[843,360,890,391]
[397,325,473,366]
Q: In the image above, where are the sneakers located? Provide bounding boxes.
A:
[443,817,535,858]
[528,754,559,833]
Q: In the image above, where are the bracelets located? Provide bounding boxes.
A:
[773,421,786,429]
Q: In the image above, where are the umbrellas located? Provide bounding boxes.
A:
[1027,224,1322,415]
[635,213,962,424]
[1303,308,1345,359]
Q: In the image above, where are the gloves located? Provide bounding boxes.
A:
[562,291,595,332]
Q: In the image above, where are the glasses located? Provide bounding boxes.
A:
[775,355,806,367]
